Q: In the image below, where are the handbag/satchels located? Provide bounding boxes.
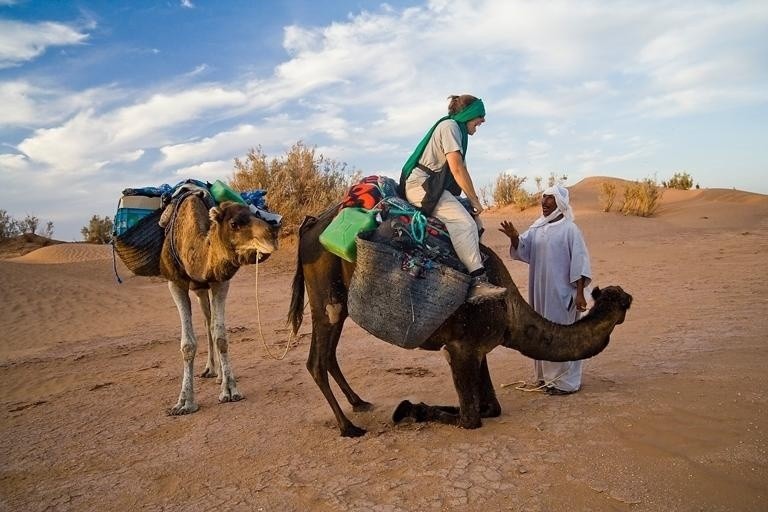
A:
[110,209,164,276]
[348,231,470,350]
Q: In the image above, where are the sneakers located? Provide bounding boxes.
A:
[467,274,508,305]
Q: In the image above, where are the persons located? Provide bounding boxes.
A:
[498,185,592,395]
[399,95,507,303]
